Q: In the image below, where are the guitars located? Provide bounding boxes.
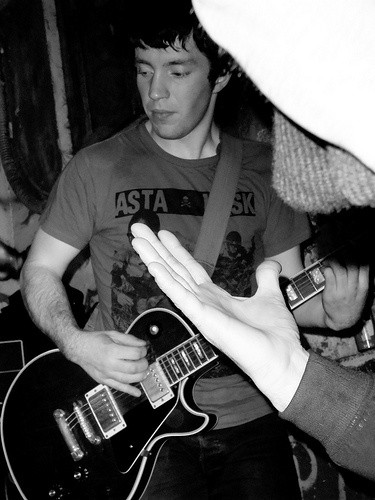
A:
[1,256,331,500]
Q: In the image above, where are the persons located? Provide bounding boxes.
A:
[128,222,375,483]
[19,0,372,500]
